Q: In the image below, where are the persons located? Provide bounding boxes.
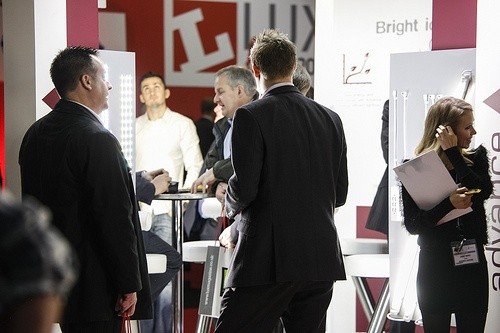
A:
[402,96,493,333]
[190,29,348,333]
[0,46,183,332]
[136,71,204,333]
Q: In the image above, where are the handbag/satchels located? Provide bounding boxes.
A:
[199,205,234,319]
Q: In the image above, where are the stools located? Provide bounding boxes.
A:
[342,236,389,333]
[183,241,222,333]
[125,253,167,333]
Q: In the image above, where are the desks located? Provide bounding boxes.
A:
[155,191,203,333]
